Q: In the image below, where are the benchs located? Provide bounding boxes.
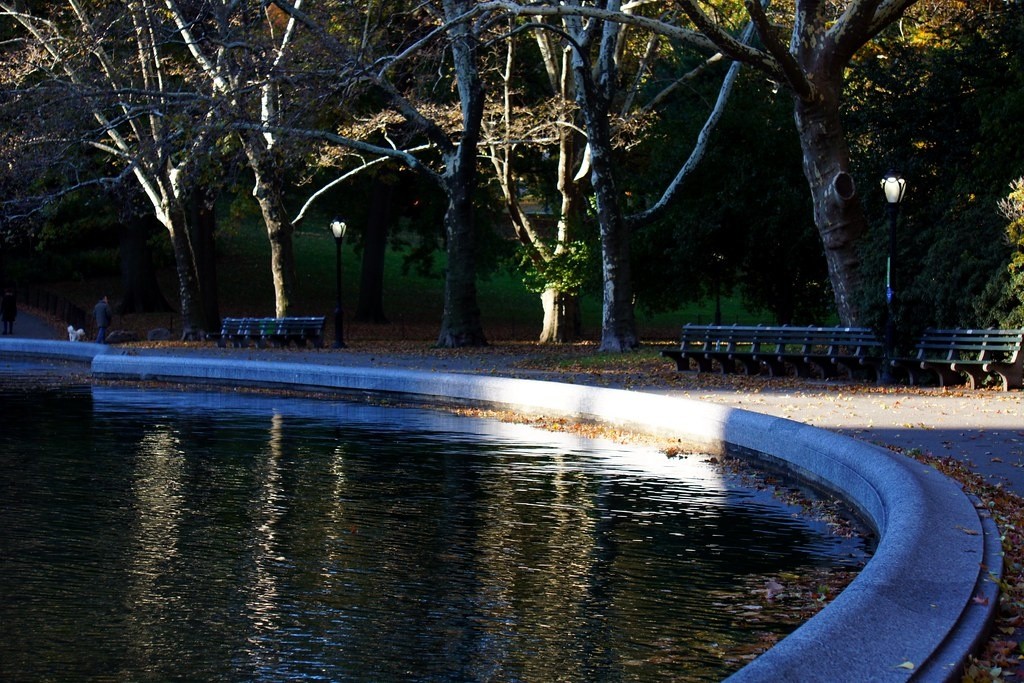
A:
[206,316,326,349]
[660,321,1024,391]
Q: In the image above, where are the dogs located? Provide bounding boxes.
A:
[67,326,85,341]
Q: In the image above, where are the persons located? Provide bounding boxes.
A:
[0,288,17,336]
[91,295,113,345]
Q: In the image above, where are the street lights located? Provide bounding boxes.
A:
[877,168,908,305]
[329,217,348,349]
